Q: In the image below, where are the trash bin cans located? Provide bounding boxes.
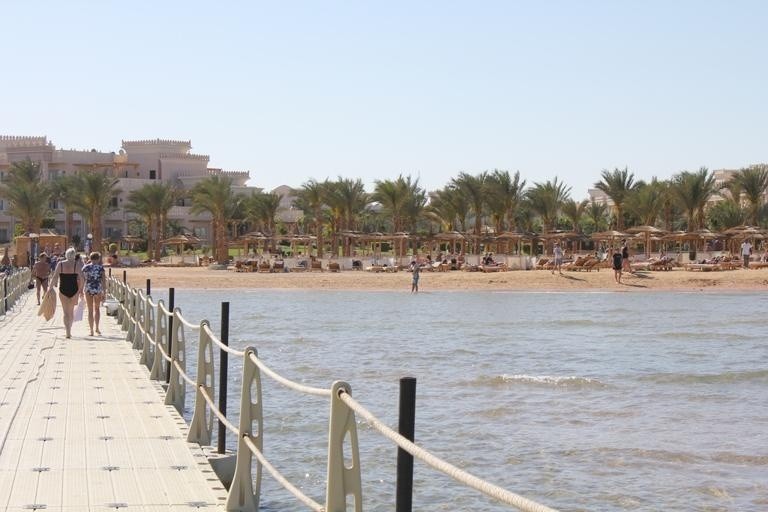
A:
[106,300,119,316]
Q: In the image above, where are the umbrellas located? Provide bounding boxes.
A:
[158,231,204,263]
[118,233,146,254]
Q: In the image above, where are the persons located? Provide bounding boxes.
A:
[552,243,564,275]
[568,244,613,273]
[406,248,465,272]
[481,252,489,265]
[0,241,153,306]
[760,249,768,263]
[619,238,632,274]
[233,246,312,273]
[612,248,623,284]
[740,238,753,269]
[486,252,498,266]
[79,251,107,336]
[697,254,739,267]
[410,260,420,293]
[47,246,84,339]
[647,249,668,265]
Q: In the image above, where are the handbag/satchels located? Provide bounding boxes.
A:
[28,282,35,289]
